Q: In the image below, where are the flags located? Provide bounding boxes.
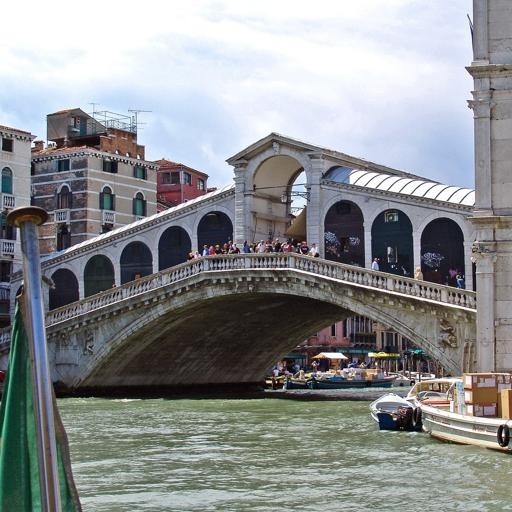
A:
[1,276,84,511]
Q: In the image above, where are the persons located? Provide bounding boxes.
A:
[281,359,301,378]
[371,258,380,281]
[312,360,320,373]
[338,359,375,370]
[413,267,424,288]
[188,238,320,270]
[272,366,279,377]
[455,274,464,289]
[455,271,464,288]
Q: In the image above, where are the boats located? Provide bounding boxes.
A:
[368,391,415,432]
[264,367,436,390]
[407,371,512,453]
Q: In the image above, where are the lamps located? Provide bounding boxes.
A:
[280,191,310,204]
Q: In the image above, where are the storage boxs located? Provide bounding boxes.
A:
[454,371,512,420]
